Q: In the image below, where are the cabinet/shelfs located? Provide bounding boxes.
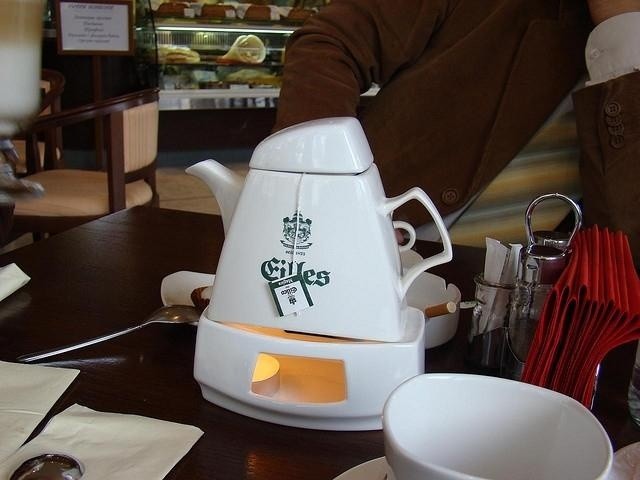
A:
[134,0,383,153]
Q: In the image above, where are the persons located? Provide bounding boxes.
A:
[270,0,640,248]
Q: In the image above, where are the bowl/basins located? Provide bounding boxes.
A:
[381,373,613,479]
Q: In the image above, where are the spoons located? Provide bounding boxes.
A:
[8,452,85,480]
[17,306,200,363]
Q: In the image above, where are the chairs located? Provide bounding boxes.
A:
[1,69,163,241]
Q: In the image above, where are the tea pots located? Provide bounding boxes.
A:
[185,114,461,341]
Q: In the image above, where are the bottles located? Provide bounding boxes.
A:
[508,193,583,364]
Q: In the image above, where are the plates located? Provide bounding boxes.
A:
[160,269,216,326]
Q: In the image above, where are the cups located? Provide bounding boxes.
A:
[470,274,516,345]
